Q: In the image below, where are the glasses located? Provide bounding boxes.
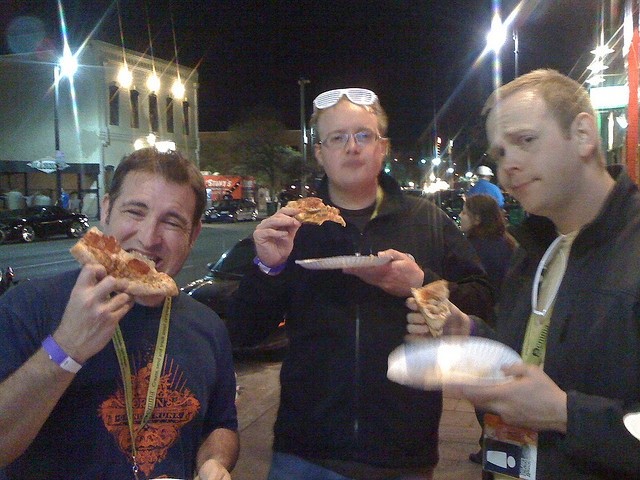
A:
[318,130,382,148]
[531,233,568,317]
[312,88,378,114]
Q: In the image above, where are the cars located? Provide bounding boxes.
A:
[178,235,287,353]
[0,206,90,243]
[204,198,259,222]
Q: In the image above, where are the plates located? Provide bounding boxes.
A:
[295,255,394,271]
[386,334,524,392]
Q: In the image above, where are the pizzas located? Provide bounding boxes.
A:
[410,280,450,337]
[284,196,346,227]
[69,225,179,299]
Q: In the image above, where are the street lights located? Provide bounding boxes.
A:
[51,54,79,206]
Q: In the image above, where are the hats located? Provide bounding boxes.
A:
[477,165,494,176]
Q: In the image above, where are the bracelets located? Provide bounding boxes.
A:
[252,253,288,277]
[39,333,83,374]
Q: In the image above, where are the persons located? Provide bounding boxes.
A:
[467,164,506,211]
[404,67,640,480]
[177,88,491,480]
[53,187,71,209]
[457,193,519,465]
[7,183,23,210]
[0,146,240,480]
[33,187,51,206]
